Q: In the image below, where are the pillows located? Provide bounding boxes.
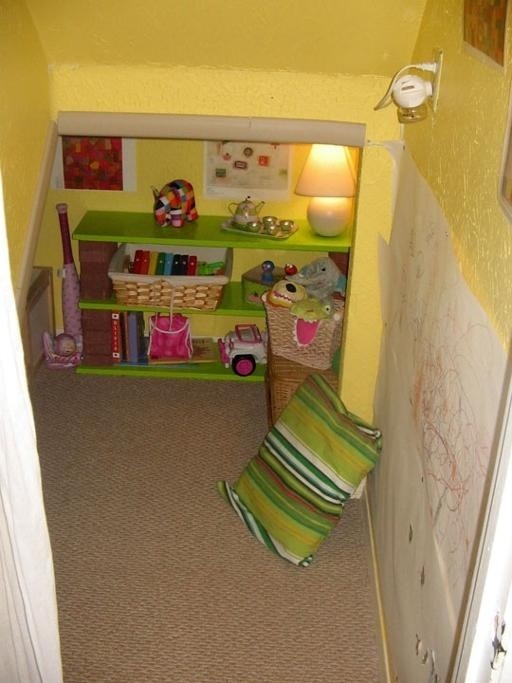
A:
[216,374,385,570]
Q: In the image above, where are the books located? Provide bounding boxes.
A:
[109,310,144,363]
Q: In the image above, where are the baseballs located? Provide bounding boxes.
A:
[54,334,75,356]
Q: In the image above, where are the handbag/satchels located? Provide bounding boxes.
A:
[146,316,193,360]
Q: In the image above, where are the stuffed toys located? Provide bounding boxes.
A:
[150,177,200,228]
[270,258,347,350]
[122,248,225,277]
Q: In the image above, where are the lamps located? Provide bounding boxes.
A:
[293,140,358,239]
[372,45,447,125]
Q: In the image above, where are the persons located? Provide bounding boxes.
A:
[259,261,274,282]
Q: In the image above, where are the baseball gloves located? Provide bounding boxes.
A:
[42,331,80,367]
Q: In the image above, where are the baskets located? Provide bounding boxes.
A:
[261,290,344,369]
[108,242,234,312]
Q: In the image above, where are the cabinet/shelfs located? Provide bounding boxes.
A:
[67,206,352,388]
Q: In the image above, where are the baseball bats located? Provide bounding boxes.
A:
[56,203,83,336]
